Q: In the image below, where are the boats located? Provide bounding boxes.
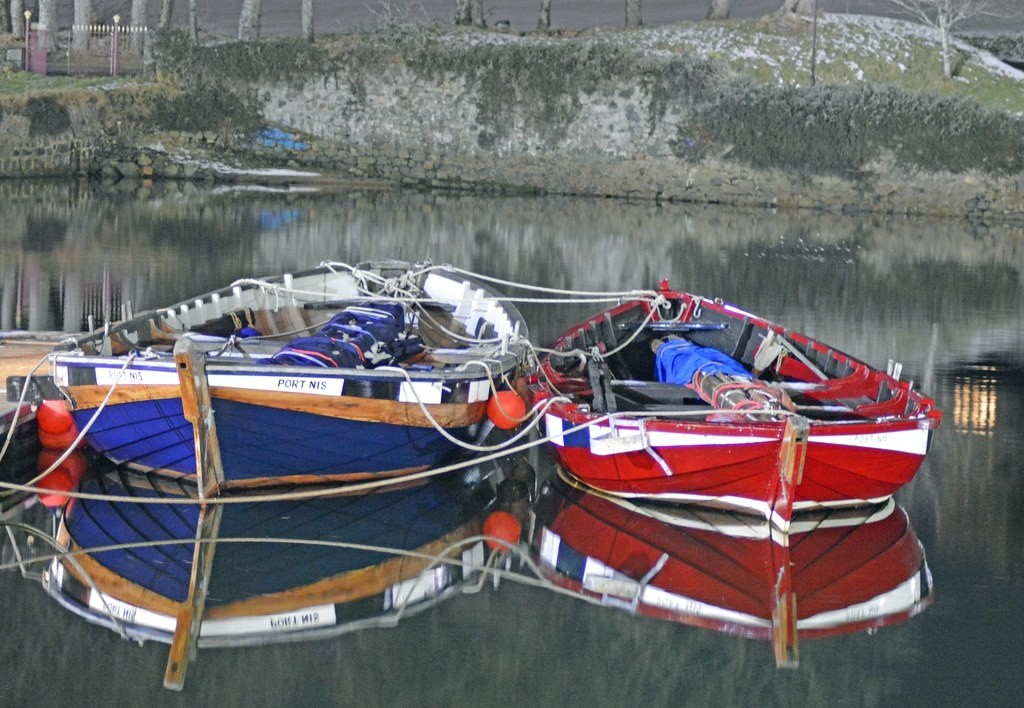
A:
[1,451,533,693]
[0,330,95,459]
[527,280,943,535]
[518,463,936,672]
[37,256,533,503]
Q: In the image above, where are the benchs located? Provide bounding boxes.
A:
[175,333,493,365]
